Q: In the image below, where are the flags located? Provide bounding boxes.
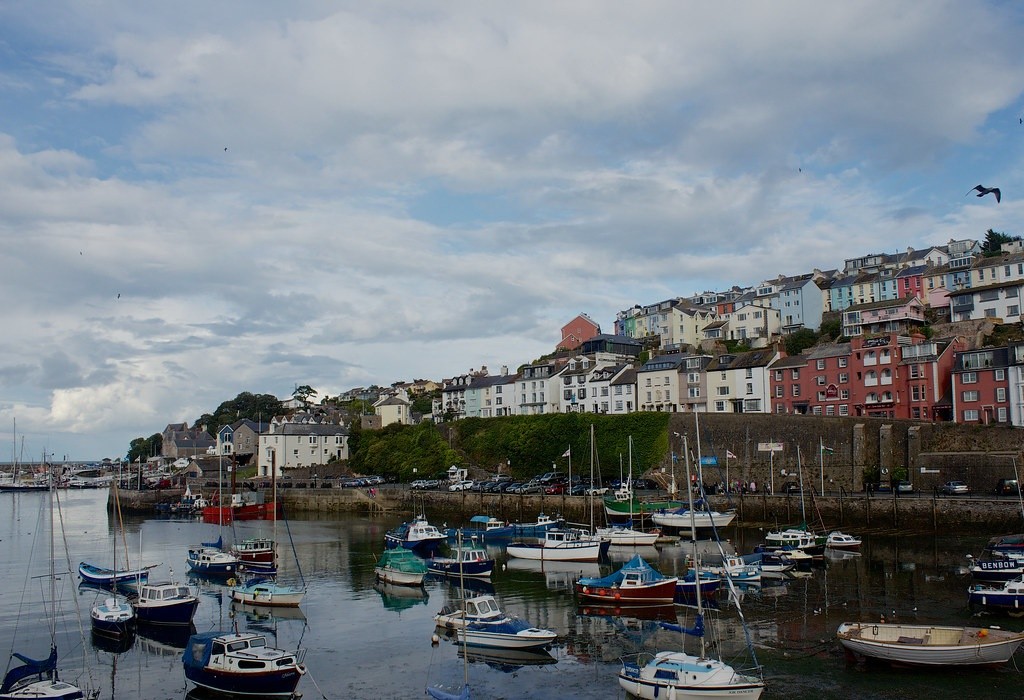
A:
[134,455,140,462]
[562,449,570,457]
[822,446,833,455]
[728,450,736,458]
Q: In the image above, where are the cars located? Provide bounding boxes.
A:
[943,481,968,495]
[899,480,913,494]
[375,405,862,610]
[782,481,803,493]
[341,475,399,487]
[410,480,440,490]
[449,474,658,496]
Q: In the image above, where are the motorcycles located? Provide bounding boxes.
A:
[765,483,772,495]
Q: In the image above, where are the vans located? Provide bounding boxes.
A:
[541,472,565,485]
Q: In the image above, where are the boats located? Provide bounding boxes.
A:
[434,595,558,649]
[155,486,280,517]
[78,561,200,643]
[187,451,282,574]
[618,437,766,700]
[837,621,1024,672]
[229,576,305,607]
[182,632,309,700]
[968,534,1024,612]
[1,462,99,700]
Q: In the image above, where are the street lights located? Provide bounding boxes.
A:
[314,473,317,488]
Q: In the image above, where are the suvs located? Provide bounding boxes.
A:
[636,479,658,490]
[997,479,1020,496]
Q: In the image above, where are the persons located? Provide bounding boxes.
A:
[368,488,376,499]
[690,479,771,495]
[438,479,451,487]
[966,555,984,569]
